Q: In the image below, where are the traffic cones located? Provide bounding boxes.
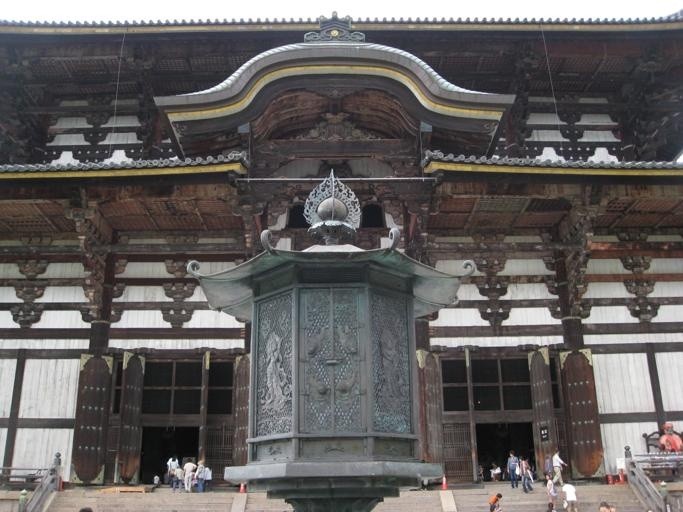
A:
[443,474,448,490]
[240,482,245,493]
[617,470,626,484]
[608,475,614,484]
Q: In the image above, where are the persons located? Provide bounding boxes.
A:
[203,462,212,492]
[169,464,183,493]
[182,457,197,494]
[477,447,578,512]
[193,458,205,493]
[597,501,616,511]
[166,455,179,488]
[47,468,63,492]
